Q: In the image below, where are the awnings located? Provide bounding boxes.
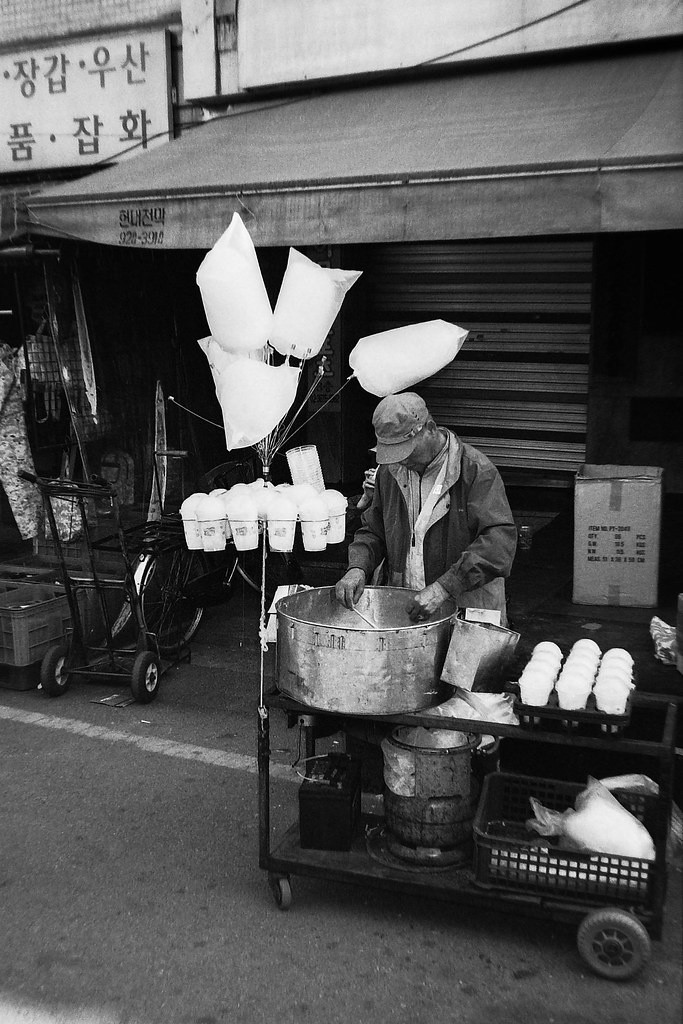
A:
[26,46,683,250]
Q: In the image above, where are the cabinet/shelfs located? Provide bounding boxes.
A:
[259,651,683,939]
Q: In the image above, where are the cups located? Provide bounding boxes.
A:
[518,639,634,733]
[180,445,348,552]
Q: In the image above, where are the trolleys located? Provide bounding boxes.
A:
[17,468,195,704]
[255,684,683,980]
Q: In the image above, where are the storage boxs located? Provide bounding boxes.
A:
[0,583,95,691]
[469,772,662,911]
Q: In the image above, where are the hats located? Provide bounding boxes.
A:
[372,392,433,464]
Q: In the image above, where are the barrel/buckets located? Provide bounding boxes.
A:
[275,585,458,716]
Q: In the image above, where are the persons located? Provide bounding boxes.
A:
[335,392,518,624]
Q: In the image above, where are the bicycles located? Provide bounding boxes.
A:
[88,456,302,659]
